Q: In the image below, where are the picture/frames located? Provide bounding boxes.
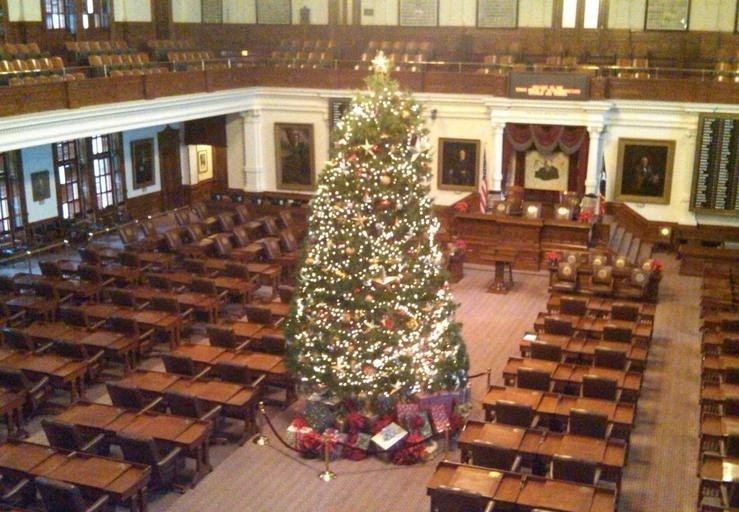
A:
[274,122,318,192]
[31,169,51,202]
[610,136,678,207]
[197,149,209,174]
[436,136,482,194]
[130,137,156,190]
